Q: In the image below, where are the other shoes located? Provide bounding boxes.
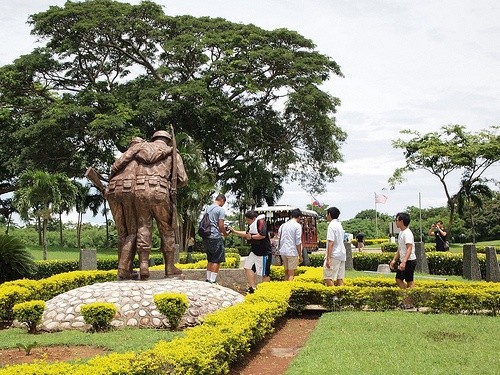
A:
[246,287,255,293]
[206,279,220,286]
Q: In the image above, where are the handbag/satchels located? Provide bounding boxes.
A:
[273,250,283,266]
[443,240,450,251]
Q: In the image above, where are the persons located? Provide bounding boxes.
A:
[356,231,364,252]
[390,212,417,309]
[228,211,273,295]
[323,207,347,309]
[106,130,188,281]
[278,208,302,281]
[203,194,233,286]
[429,220,449,252]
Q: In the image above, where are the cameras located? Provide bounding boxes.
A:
[433,225,437,227]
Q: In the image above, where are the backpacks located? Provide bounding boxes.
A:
[271,239,277,255]
[198,205,218,238]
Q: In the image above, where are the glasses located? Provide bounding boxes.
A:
[395,220,400,222]
